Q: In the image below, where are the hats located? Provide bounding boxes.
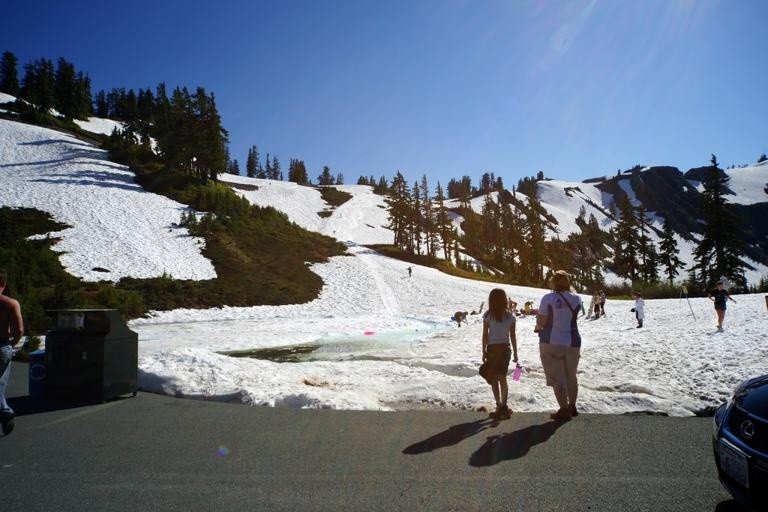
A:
[478,362,495,385]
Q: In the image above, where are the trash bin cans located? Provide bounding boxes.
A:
[46,305,139,405]
[27,349,46,400]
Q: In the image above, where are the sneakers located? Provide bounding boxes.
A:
[489,405,514,421]
[550,407,571,421]
[2,410,16,437]
[568,405,578,417]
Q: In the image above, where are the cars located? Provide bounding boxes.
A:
[710,374,767,512]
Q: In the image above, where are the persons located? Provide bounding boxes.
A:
[1,266,25,436]
[452,309,469,326]
[593,290,602,318]
[481,286,519,420]
[512,302,518,316]
[708,280,737,333]
[524,300,534,316]
[507,295,513,315]
[533,269,585,423]
[632,292,645,328]
[598,290,607,316]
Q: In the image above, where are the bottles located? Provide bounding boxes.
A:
[513,365,522,381]
